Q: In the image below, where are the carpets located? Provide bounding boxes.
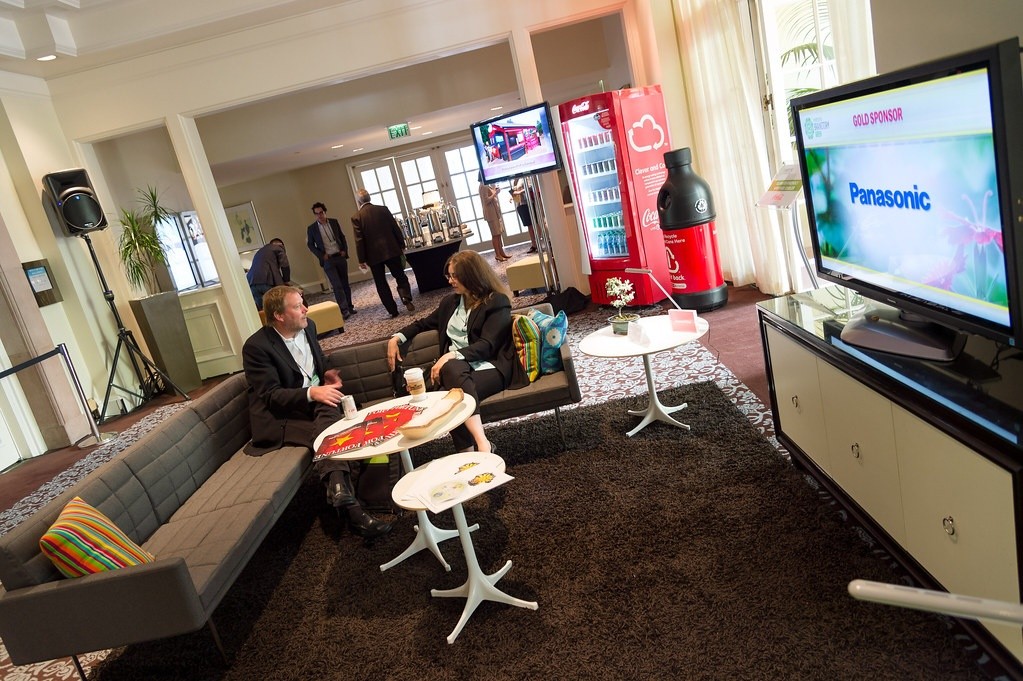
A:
[76,379,1023,681]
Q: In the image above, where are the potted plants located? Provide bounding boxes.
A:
[117,190,204,395]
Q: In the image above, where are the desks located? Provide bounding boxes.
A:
[313,390,476,572]
[580,316,709,437]
[391,452,539,644]
[401,224,474,294]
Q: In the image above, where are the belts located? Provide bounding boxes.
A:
[328,252,341,258]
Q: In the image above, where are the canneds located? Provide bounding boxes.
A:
[578,130,627,228]
[341,395,358,419]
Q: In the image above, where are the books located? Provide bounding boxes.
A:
[313,418,366,462]
[363,405,428,442]
[363,417,384,446]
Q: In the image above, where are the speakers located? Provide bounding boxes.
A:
[41,169,109,237]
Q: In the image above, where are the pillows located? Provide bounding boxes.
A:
[39,496,155,577]
[528,307,568,375]
[511,314,539,382]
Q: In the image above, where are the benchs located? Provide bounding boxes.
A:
[0,303,581,681]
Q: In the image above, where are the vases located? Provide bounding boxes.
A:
[607,313,640,335]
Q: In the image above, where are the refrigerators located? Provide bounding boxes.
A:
[558,85,688,307]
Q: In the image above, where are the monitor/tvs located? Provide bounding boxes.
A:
[469,102,562,186]
[790,36,1023,361]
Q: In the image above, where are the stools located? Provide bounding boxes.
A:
[306,301,345,335]
[507,253,557,297]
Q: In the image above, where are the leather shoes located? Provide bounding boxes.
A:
[325,481,356,507]
[345,511,392,536]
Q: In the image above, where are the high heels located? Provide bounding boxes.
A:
[495,256,507,262]
[500,252,512,258]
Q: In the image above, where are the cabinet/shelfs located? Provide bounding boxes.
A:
[760,322,1023,681]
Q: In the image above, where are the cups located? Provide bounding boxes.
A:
[403,367,427,400]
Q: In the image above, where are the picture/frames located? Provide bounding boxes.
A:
[224,200,266,256]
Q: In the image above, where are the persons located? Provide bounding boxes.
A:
[486,140,493,163]
[242,286,392,538]
[249,238,291,310]
[478,172,513,262]
[351,189,415,318]
[307,202,358,319]
[388,250,531,454]
[510,176,552,253]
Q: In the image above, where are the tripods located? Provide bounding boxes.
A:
[81,232,193,425]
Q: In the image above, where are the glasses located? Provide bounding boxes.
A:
[314,210,324,216]
[444,272,464,281]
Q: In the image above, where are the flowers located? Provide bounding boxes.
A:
[605,278,634,317]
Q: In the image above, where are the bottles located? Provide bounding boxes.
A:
[657,148,730,317]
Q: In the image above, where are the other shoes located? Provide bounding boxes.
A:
[401,297,415,311]
[392,311,399,318]
[343,310,350,320]
[348,308,357,314]
[474,441,497,453]
[527,246,537,253]
